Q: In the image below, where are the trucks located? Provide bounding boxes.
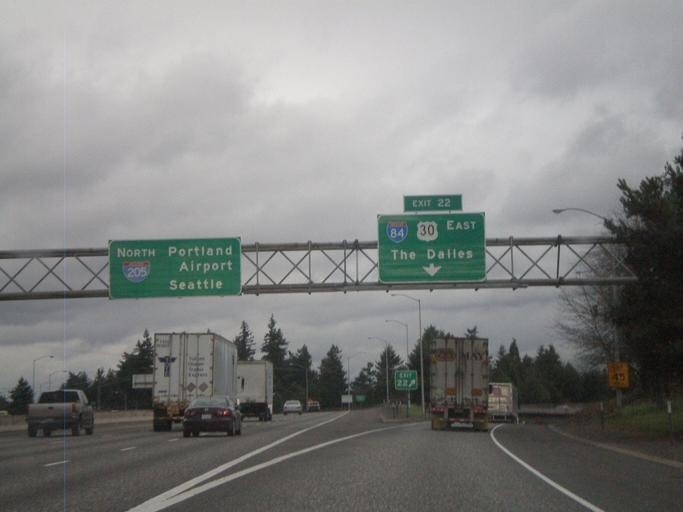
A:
[235,359,274,422]
[488,382,519,424]
[151,332,238,432]
[427,337,489,432]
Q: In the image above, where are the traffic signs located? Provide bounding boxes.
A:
[401,195,462,211]
[108,237,241,298]
[377,213,487,283]
[609,363,629,387]
[394,370,417,391]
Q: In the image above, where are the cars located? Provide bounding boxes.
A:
[282,400,302,414]
[182,395,241,437]
[306,400,319,411]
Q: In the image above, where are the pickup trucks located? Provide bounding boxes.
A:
[24,388,94,436]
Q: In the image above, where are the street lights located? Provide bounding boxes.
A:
[32,355,53,395]
[289,363,309,402]
[346,351,366,411]
[49,369,66,391]
[385,319,411,418]
[367,336,389,406]
[554,207,621,412]
[390,293,425,419]
